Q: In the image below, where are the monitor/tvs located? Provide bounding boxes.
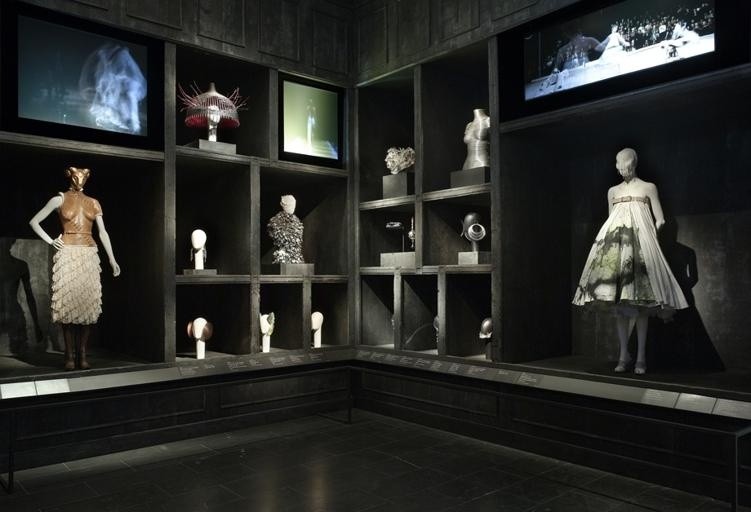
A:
[279,71,344,169]
[0,0,164,153]
[497,0,727,124]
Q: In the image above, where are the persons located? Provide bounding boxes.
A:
[461,107,488,169]
[599,23,631,58]
[29,167,122,371]
[305,96,318,149]
[572,147,689,374]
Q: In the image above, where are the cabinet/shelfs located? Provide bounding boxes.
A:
[2,1,352,405]
[353,1,750,424]
